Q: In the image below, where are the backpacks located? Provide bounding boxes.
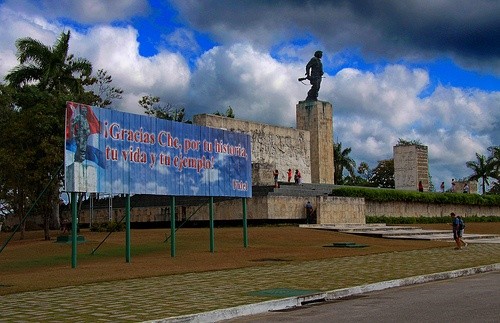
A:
[454,217,465,230]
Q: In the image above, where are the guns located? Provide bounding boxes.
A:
[298,76,325,81]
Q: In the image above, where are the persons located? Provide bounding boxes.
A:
[451,179,456,193]
[447,212,468,250]
[69,107,90,162]
[287,168,291,183]
[306,51,324,101]
[304,202,313,219]
[294,169,302,187]
[419,180,424,193]
[463,184,469,194]
[440,182,445,193]
[273,169,279,188]
